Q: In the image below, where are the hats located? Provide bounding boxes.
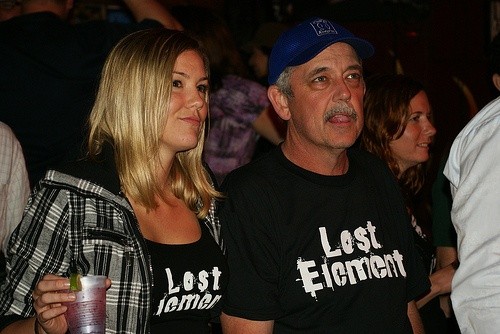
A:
[268,17,375,84]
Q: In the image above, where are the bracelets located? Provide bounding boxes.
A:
[34,318,39,334]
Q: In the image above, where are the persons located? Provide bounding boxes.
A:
[219,18,432,334]
[0,30,226,334]
[0,0,179,188]
[362,35,500,334]
[193,21,286,185]
[0,122,31,248]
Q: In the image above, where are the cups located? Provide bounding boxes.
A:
[58,275,107,334]
